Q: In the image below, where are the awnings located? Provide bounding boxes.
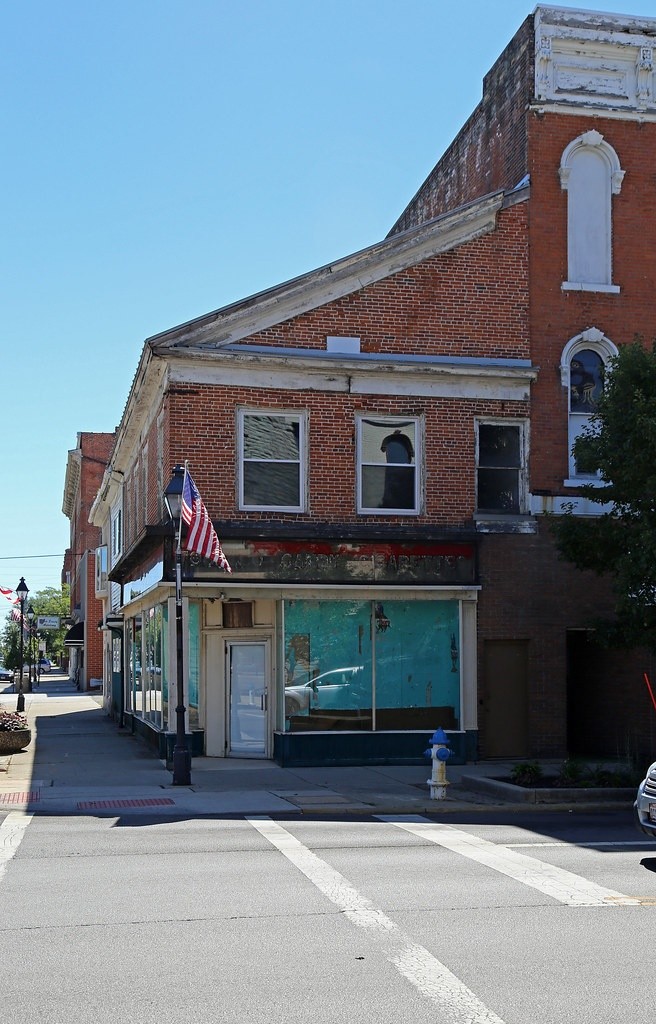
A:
[64,622,84,647]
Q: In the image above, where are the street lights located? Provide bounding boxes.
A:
[162,463,193,783]
[27,605,40,692]
[14,576,30,712]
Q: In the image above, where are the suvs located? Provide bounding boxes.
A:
[634,761,656,839]
[284,665,367,714]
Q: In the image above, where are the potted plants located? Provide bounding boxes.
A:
[0,711,32,751]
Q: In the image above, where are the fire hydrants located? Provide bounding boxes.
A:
[424,726,455,801]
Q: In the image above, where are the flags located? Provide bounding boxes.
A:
[0,586,22,604]
[179,467,232,574]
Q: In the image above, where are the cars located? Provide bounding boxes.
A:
[0,659,15,683]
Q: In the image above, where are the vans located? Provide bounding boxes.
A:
[21,657,51,674]
